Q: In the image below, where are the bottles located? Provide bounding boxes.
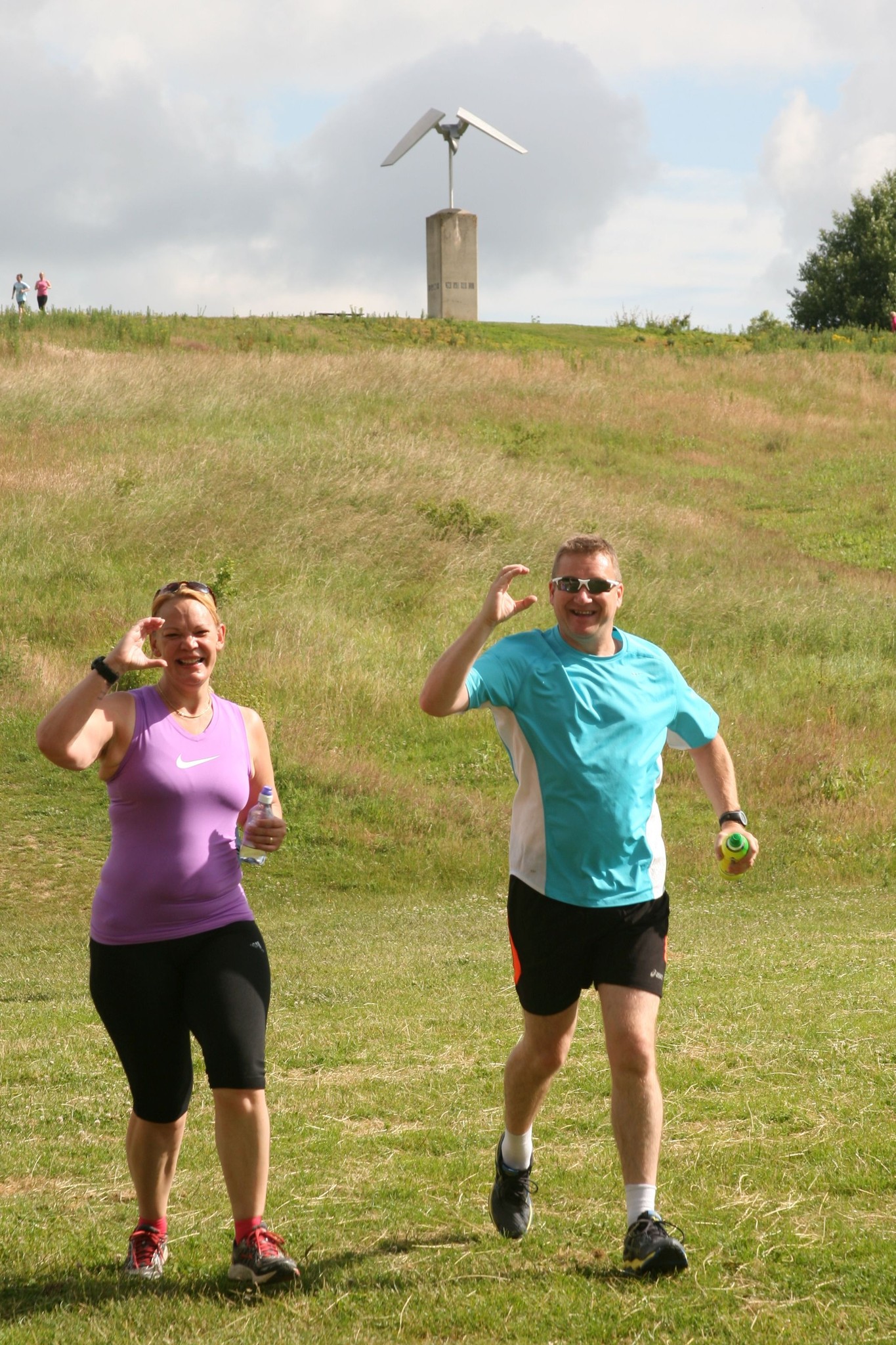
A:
[718,832,749,881]
[239,786,274,867]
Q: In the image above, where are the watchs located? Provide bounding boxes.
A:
[719,810,747,831]
[91,656,119,683]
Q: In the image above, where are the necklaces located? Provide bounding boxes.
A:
[157,681,211,718]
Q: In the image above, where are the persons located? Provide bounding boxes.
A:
[420,535,760,1278]
[37,581,301,1287]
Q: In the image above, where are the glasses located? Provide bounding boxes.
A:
[552,576,619,594]
[152,581,217,617]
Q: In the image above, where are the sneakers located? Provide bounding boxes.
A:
[621,1210,688,1275]
[488,1131,539,1243]
[227,1222,300,1284]
[123,1225,169,1279]
